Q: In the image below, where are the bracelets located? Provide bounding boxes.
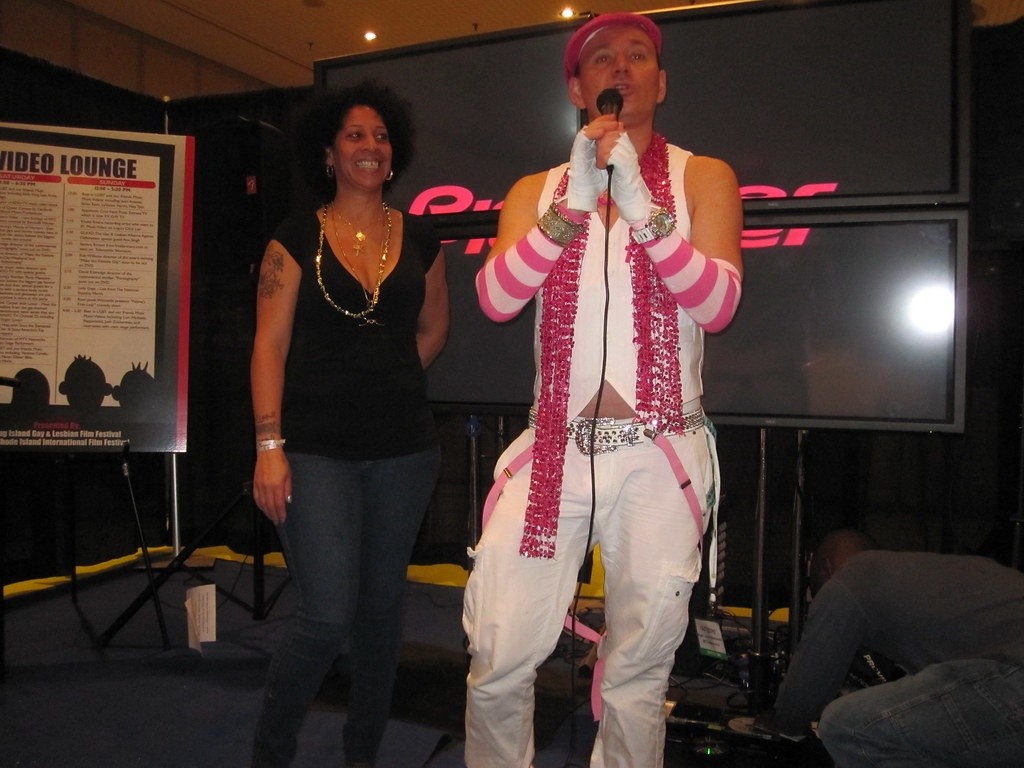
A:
[538,204,583,247]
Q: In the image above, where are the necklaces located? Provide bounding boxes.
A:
[315,197,392,318]
[256,439,286,451]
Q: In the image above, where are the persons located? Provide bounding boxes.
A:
[765,526,1024,768]
[462,12,743,768]
[246,86,449,768]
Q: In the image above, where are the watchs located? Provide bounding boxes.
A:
[631,206,676,245]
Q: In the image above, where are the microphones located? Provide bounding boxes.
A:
[596,90,624,177]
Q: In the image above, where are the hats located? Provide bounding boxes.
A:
[564,12,661,80]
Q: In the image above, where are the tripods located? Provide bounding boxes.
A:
[98,431,291,647]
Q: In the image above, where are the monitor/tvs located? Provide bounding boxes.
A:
[310,0,969,436]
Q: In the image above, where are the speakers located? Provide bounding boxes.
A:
[195,116,294,274]
